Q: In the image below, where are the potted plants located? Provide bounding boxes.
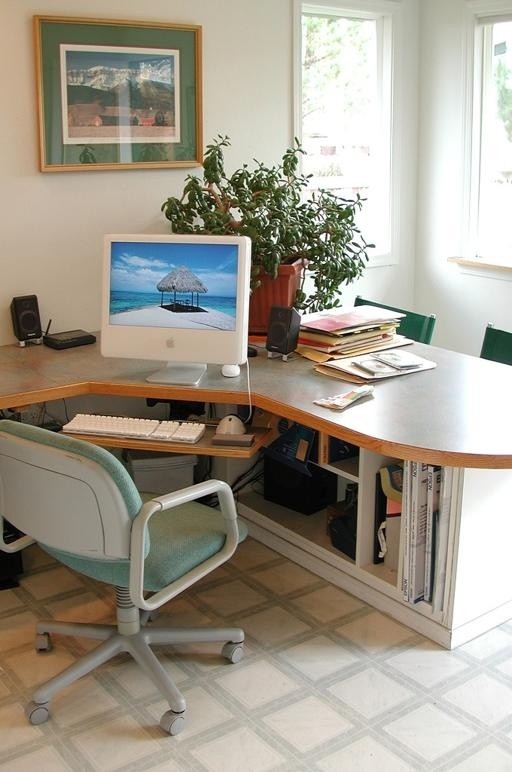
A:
[160,131,377,335]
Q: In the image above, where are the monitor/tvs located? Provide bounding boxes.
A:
[100,233,253,386]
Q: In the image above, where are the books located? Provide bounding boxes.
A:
[396,458,451,612]
[293,303,437,385]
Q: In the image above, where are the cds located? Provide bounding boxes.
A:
[379,354,401,362]
[361,360,398,373]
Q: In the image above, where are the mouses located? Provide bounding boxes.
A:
[221,364,241,378]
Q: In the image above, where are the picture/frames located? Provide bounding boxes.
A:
[34,14,202,173]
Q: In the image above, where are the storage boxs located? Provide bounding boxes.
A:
[124,444,201,504]
[261,452,337,517]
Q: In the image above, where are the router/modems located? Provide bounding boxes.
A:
[43,319,96,350]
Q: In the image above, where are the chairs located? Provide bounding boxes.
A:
[480,320,511,366]
[354,295,436,344]
[2,419,249,735]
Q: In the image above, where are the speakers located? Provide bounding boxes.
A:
[266,304,302,356]
[10,294,42,342]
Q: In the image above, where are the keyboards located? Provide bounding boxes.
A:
[62,413,206,444]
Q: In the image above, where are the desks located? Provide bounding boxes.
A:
[1,328,512,651]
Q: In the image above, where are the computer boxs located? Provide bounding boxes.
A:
[263,417,342,517]
